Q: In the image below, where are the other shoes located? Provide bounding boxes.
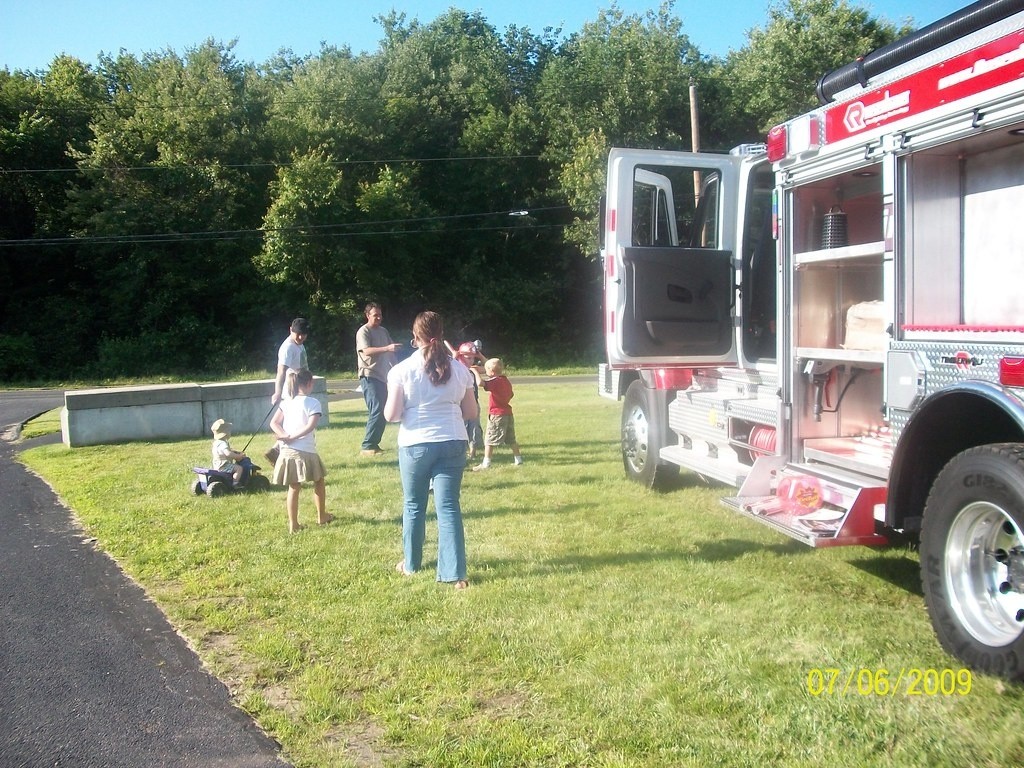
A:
[453,580,468,589]
[396,559,412,576]
[265,448,280,467]
[473,464,490,472]
[326,513,333,522]
[362,447,385,455]
[232,482,245,489]
[294,522,308,532]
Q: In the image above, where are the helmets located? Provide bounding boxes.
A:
[459,342,479,355]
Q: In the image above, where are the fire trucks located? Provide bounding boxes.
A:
[595,0,1024,680]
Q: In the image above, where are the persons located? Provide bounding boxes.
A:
[211,419,246,490]
[384,311,479,592]
[270,368,334,533]
[450,341,489,463]
[354,302,404,457]
[468,357,522,471]
[265,318,310,468]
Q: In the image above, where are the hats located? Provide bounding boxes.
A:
[211,418,233,439]
[291,318,308,334]
